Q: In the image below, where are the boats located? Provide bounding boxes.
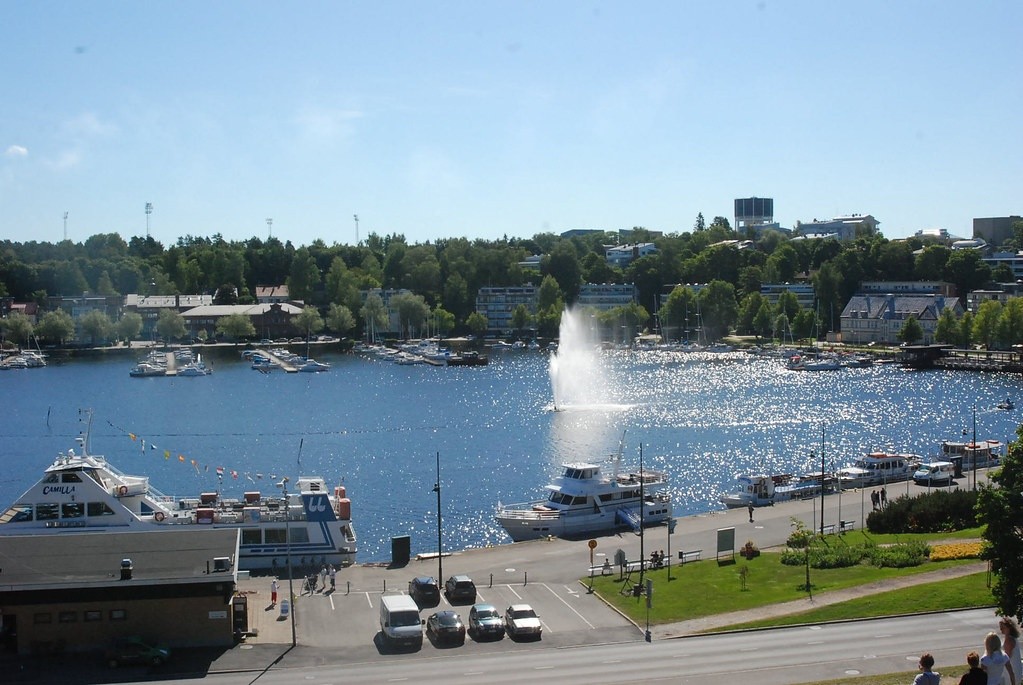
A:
[239,324,330,372]
[492,328,557,352]
[744,345,762,355]
[936,434,1007,470]
[0,328,51,370]
[130,328,168,376]
[493,428,673,542]
[719,447,926,508]
[175,346,208,378]
[999,397,1016,410]
[0,407,359,571]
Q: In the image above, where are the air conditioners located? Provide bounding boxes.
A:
[214,557,230,570]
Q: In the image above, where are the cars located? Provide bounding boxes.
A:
[469,604,505,640]
[430,610,466,643]
[304,335,319,341]
[261,339,273,344]
[102,631,174,670]
[275,337,289,342]
[182,337,217,345]
[290,337,302,342]
[507,605,543,638]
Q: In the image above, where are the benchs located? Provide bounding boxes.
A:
[816,525,835,535]
[588,564,615,577]
[836,521,855,531]
[683,550,703,562]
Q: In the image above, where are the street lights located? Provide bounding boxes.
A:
[636,442,646,573]
[808,421,825,537]
[663,515,676,582]
[433,450,446,590]
[963,405,978,490]
[282,477,301,648]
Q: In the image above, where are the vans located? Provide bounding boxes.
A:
[915,461,956,485]
[380,592,425,647]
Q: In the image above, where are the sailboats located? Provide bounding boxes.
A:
[351,314,490,366]
[778,296,877,372]
[599,298,734,354]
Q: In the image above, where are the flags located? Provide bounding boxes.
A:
[130,433,169,460]
[244,472,276,483]
[177,455,238,480]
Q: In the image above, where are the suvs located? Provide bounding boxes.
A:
[445,575,478,604]
[409,576,443,607]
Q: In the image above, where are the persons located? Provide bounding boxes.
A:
[305,563,336,591]
[748,501,754,522]
[998,617,1023,685]
[271,580,280,605]
[650,550,664,568]
[913,653,940,685]
[880,488,887,508]
[601,558,610,574]
[980,632,1015,685]
[959,651,988,685]
[871,490,880,510]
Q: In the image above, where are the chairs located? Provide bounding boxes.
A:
[626,555,673,572]
